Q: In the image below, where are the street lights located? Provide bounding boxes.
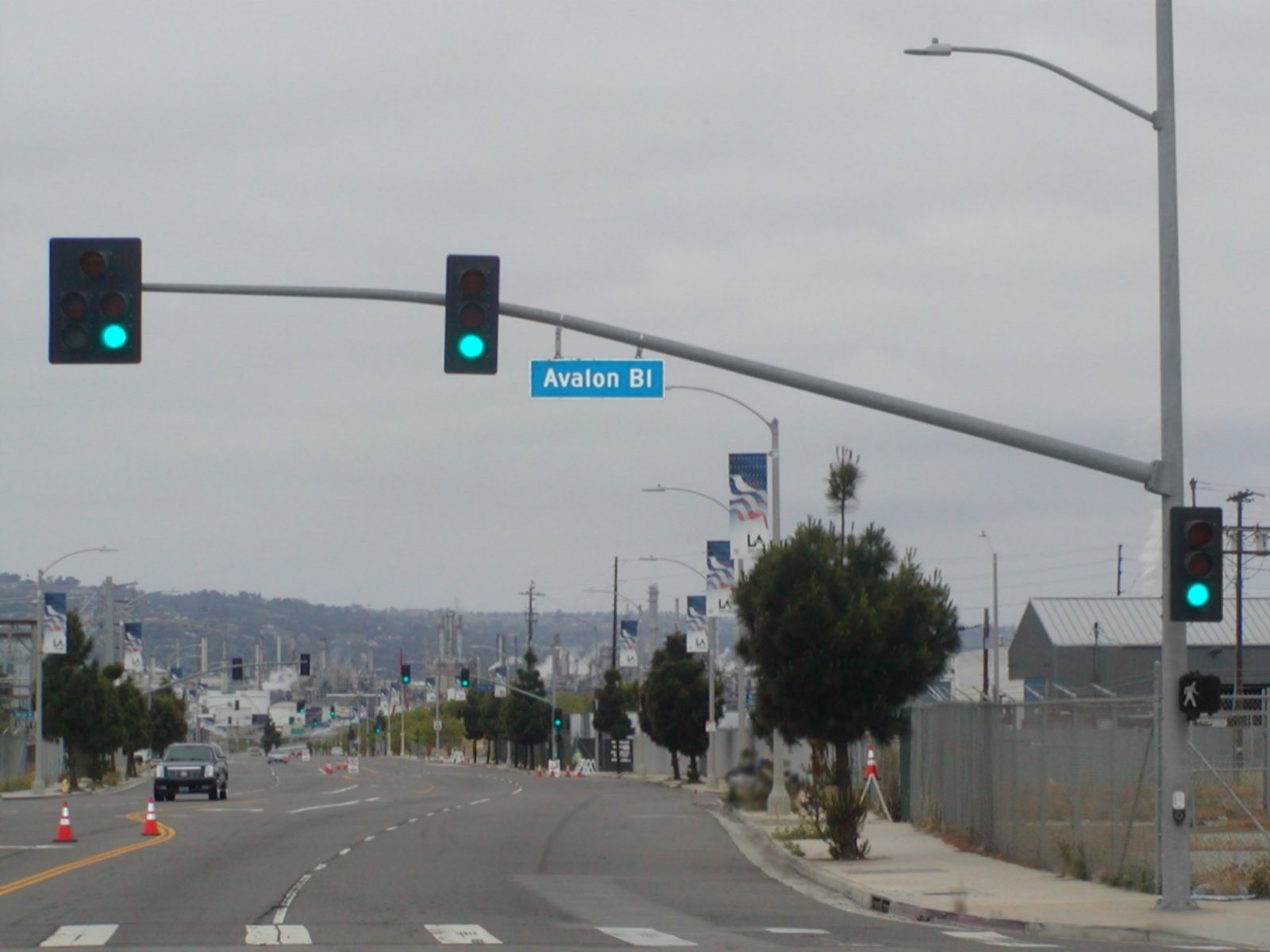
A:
[641,558,717,789]
[667,384,789,817]
[905,45,1190,913]
[644,487,746,771]
[31,549,119,794]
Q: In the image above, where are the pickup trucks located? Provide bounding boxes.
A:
[154,741,228,802]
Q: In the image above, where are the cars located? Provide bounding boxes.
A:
[268,751,289,763]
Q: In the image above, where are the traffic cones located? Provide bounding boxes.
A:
[52,802,78,842]
[140,796,161,835]
[324,761,347,774]
[534,764,583,777]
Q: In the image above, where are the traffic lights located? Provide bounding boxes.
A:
[401,665,411,683]
[49,237,142,365]
[1170,506,1223,623]
[554,708,562,728]
[461,668,470,688]
[289,702,317,727]
[1179,671,1221,715]
[444,254,500,375]
[330,707,334,717]
